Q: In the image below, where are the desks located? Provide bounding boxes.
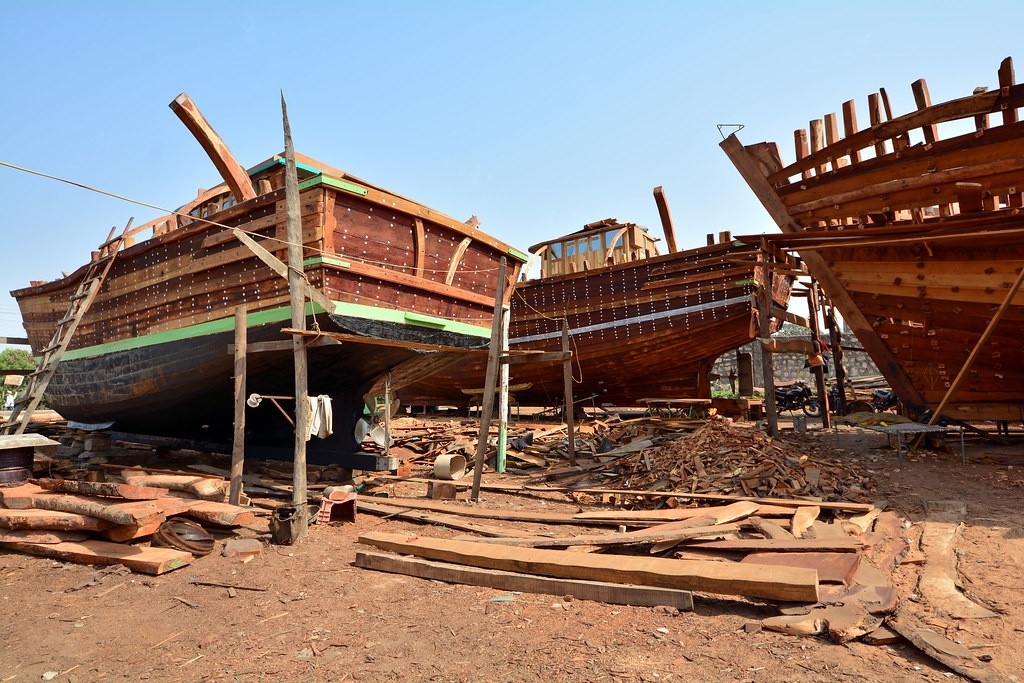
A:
[468,401,520,423]
[863,422,965,466]
[636,398,712,418]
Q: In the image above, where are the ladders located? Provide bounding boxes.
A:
[1,218,139,434]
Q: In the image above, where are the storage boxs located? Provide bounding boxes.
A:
[428,481,457,500]
[316,492,357,524]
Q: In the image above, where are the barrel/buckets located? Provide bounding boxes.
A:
[433,454,467,480]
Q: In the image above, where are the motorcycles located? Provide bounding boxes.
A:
[871,389,903,413]
[761,376,822,419]
[823,378,852,415]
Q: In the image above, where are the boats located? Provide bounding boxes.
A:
[718,56,1024,425]
[9,90,530,442]
[512,217,796,409]
